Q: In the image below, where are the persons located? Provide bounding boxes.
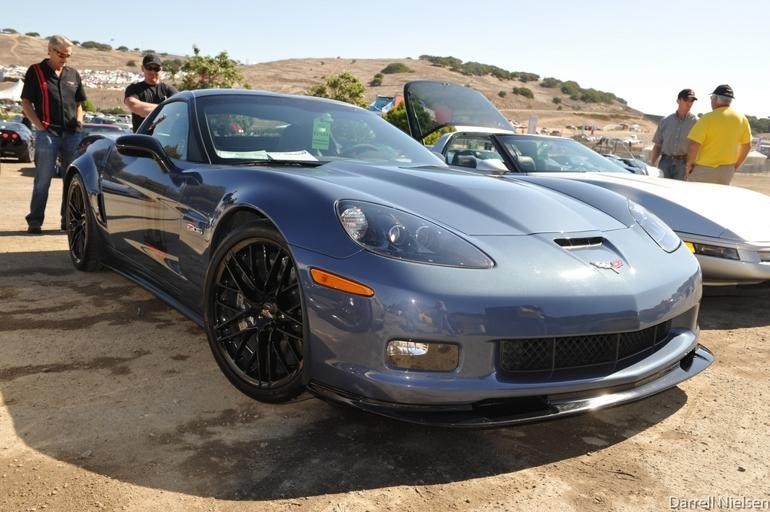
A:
[20,35,87,234]
[123,53,177,134]
[685,84,753,185]
[650,89,698,179]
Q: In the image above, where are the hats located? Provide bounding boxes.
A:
[142,54,162,66]
[708,84,735,99]
[678,88,698,101]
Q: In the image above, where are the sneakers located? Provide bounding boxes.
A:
[28,226,42,234]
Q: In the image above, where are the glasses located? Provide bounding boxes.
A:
[144,64,160,72]
[683,97,694,103]
[54,48,69,58]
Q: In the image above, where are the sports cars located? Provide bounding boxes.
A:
[61,87,716,431]
[402,79,770,289]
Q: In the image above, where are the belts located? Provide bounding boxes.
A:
[661,154,687,160]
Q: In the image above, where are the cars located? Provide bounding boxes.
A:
[0,120,36,163]
